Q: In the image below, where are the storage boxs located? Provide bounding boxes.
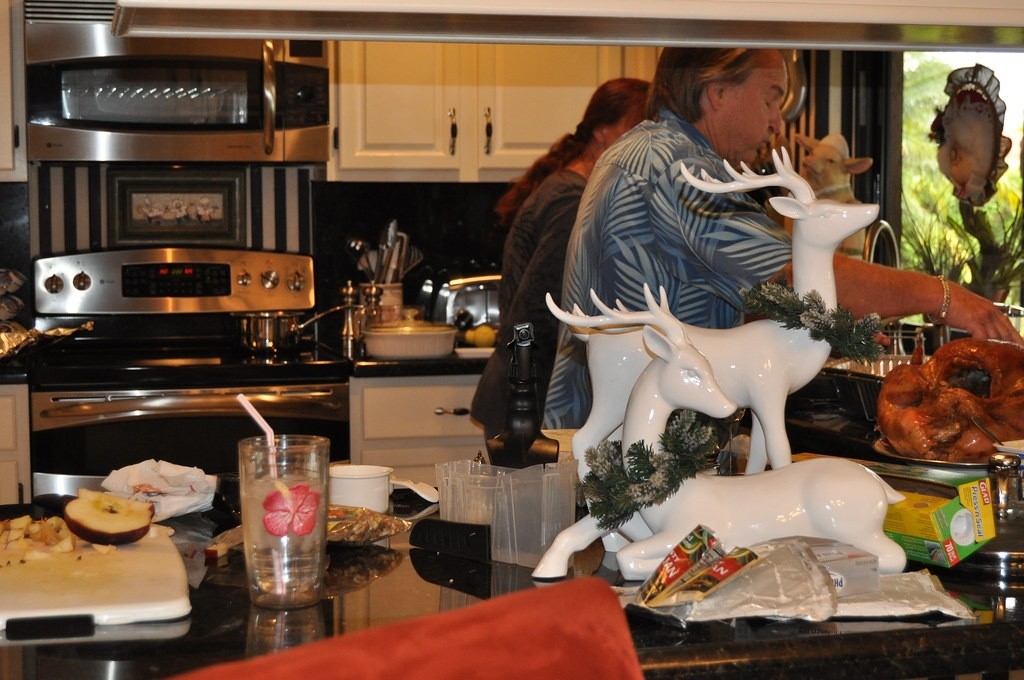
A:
[790,452,995,569]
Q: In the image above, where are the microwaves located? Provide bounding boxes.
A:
[24,1,330,161]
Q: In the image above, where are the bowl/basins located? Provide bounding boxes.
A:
[360,308,458,357]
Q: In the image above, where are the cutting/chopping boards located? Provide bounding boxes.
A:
[0,523,192,632]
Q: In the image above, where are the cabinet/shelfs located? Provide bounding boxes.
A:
[0,385,33,505]
[346,376,493,487]
[326,40,624,184]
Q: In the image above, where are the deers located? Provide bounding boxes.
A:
[528,283,906,581]
[572,147,880,554]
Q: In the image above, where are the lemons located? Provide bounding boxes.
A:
[474,325,496,346]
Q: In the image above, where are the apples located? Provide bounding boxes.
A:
[64,489,155,546]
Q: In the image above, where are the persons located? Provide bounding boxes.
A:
[541,47,1024,439]
[469,78,653,557]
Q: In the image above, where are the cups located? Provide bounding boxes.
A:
[238,434,329,607]
[359,283,402,320]
[329,465,393,513]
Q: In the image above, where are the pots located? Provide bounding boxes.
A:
[241,304,363,352]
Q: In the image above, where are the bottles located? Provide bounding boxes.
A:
[363,280,384,324]
[341,281,360,358]
[990,452,1022,520]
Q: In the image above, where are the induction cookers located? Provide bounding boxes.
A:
[31,246,354,392]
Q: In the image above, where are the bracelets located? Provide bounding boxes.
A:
[927,274,951,325]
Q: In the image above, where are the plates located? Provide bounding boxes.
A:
[873,436,988,466]
[993,440,1024,455]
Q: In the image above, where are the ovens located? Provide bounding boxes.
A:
[26,391,348,530]
